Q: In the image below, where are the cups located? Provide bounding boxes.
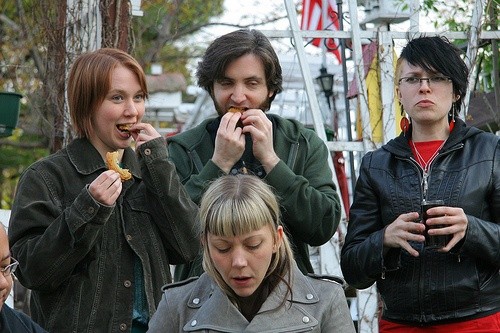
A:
[421,200,447,251]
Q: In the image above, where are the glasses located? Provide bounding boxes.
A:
[398,74,452,88]
[0,257,20,278]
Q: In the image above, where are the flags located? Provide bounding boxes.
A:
[300,0,343,64]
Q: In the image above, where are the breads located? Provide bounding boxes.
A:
[228,107,246,114]
[118,124,139,133]
[106,151,132,179]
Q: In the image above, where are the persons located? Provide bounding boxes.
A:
[165,29,342,282]
[340,36,500,333]
[7,48,200,333]
[147,174,357,333]
[0,221,50,333]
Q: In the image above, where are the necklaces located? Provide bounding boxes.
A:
[410,136,449,190]
